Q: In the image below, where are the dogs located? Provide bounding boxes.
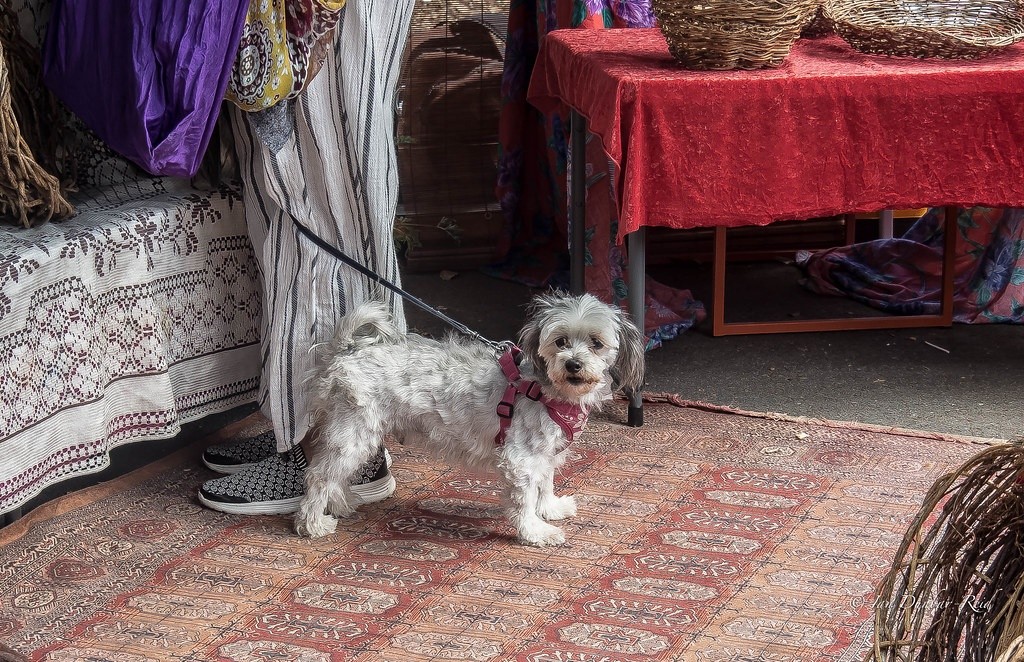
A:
[291,283,646,546]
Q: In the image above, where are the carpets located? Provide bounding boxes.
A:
[0,393,1024,662]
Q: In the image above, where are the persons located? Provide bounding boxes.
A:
[197,0,416,514]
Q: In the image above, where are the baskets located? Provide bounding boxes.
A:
[824,0,1024,63]
[652,0,803,68]
[868,438,1024,662]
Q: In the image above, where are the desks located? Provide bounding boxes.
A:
[0,150,263,522]
[527,28,1023,428]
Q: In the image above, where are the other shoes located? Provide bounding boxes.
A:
[202,430,393,476]
[198,442,397,516]
